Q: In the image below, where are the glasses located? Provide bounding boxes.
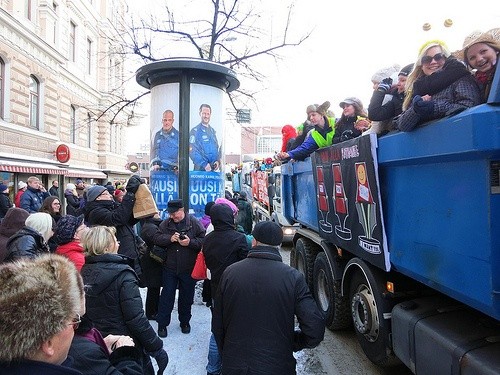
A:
[421,53,443,63]
[68,314,82,329]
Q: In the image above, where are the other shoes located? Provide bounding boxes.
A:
[158,325,167,337]
[180,323,191,334]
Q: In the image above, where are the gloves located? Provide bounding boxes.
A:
[377,77,393,92]
[154,349,168,373]
[126,177,140,197]
[413,95,433,115]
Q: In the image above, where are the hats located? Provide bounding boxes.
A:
[0,183,7,191]
[371,64,400,81]
[252,221,283,246]
[164,199,183,213]
[451,28,500,60]
[306,101,330,116]
[18,181,26,189]
[215,198,239,216]
[339,97,363,110]
[55,214,85,244]
[398,63,415,76]
[88,186,107,201]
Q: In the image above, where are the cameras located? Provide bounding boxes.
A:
[178,229,187,240]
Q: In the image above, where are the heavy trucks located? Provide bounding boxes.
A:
[280,53,500,375]
[240,165,282,224]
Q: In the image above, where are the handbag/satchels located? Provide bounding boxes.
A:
[149,247,168,264]
[132,184,158,219]
[191,251,206,279]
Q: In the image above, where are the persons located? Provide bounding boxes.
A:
[190,103,219,171]
[6,212,54,261]
[212,221,324,375]
[20,176,50,214]
[354,63,401,137]
[150,109,179,172]
[200,158,275,312]
[15,180,27,208]
[0,253,85,375]
[154,200,207,339]
[0,179,164,318]
[450,27,500,106]
[54,214,88,272]
[79,226,169,375]
[332,97,368,144]
[368,63,415,130]
[276,100,340,160]
[396,39,480,133]
[86,174,145,275]
[290,113,316,162]
[60,324,145,375]
[0,184,15,225]
[0,208,30,265]
[281,125,297,152]
